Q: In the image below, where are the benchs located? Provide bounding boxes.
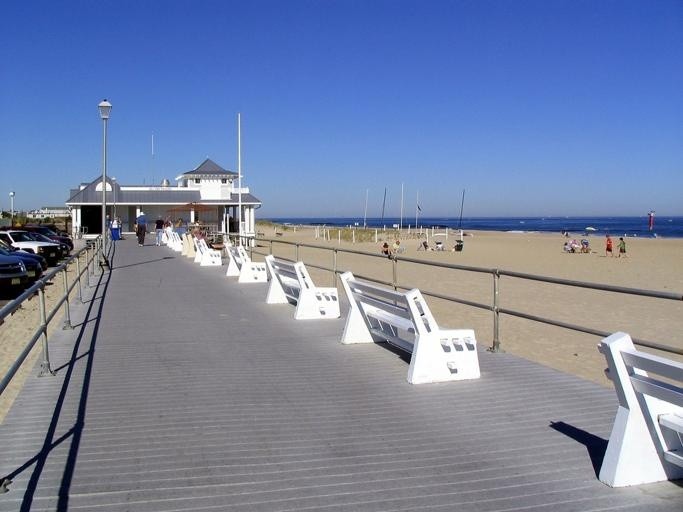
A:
[592,326,683,496]
[259,252,343,323]
[157,225,220,272]
[220,242,270,288]
[330,267,486,396]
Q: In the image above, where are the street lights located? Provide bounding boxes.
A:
[8,191,15,225]
[98,98,112,267]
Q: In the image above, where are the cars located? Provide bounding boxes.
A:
[0,221,73,298]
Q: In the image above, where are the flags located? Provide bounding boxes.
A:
[416,201,422,215]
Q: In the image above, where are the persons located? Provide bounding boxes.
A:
[616,237,628,258]
[380,243,392,255]
[392,240,406,254]
[604,234,613,257]
[560,229,591,256]
[135,211,147,247]
[105,214,122,240]
[153,216,164,246]
